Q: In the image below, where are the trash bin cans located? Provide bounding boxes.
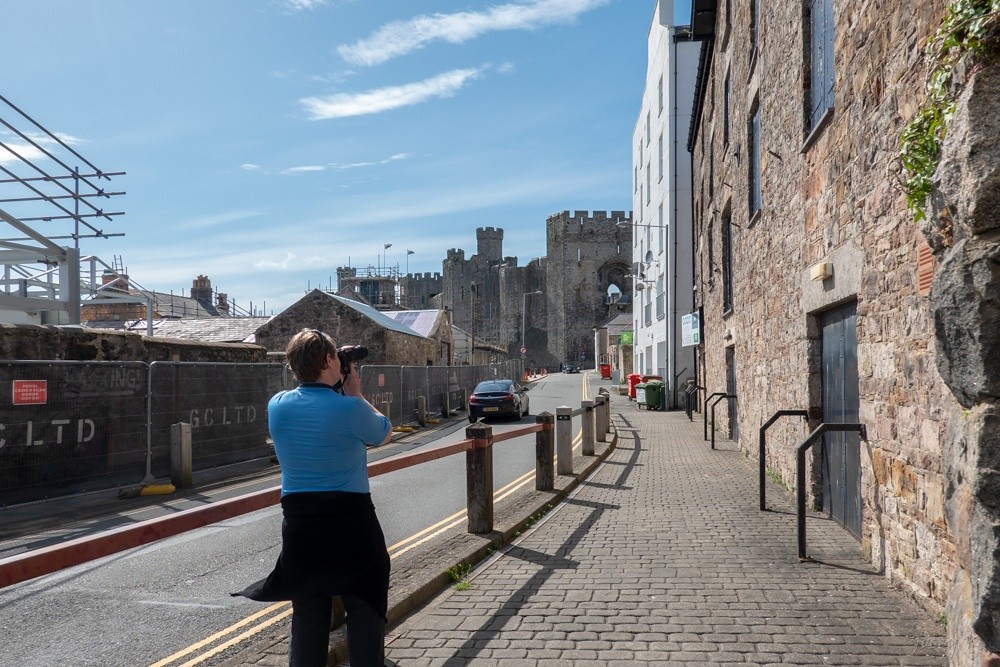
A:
[600,365,612,380]
[627,374,642,402]
[634,384,661,410]
[651,380,665,409]
[638,376,661,383]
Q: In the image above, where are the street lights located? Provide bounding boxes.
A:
[522,290,543,378]
[617,221,669,410]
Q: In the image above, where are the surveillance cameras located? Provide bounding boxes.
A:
[692,285,697,292]
[714,267,720,273]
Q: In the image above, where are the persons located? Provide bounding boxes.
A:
[231,328,392,667]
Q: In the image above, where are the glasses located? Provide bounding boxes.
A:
[306,330,325,350]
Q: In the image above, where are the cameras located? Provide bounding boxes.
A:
[337,345,369,374]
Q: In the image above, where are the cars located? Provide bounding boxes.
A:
[468,380,531,423]
[562,362,580,374]
